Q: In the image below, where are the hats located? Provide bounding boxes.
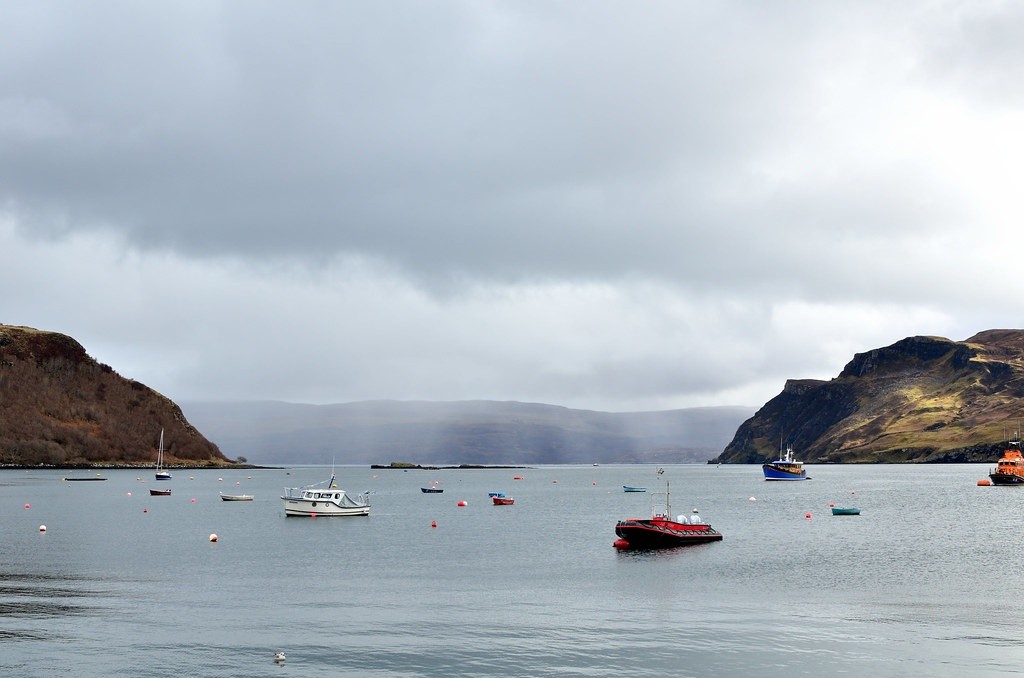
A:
[693,508,698,512]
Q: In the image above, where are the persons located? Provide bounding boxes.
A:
[995,468,1015,475]
[676,515,687,524]
[690,508,704,524]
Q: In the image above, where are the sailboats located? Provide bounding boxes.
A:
[156,428,170,479]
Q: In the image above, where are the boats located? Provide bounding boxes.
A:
[762,423,808,481]
[280,456,373,516]
[494,498,514,506]
[220,494,255,501]
[489,492,505,497]
[150,489,169,496]
[988,424,1023,486]
[623,485,647,492]
[421,487,443,493]
[832,507,861,516]
[613,468,722,549]
